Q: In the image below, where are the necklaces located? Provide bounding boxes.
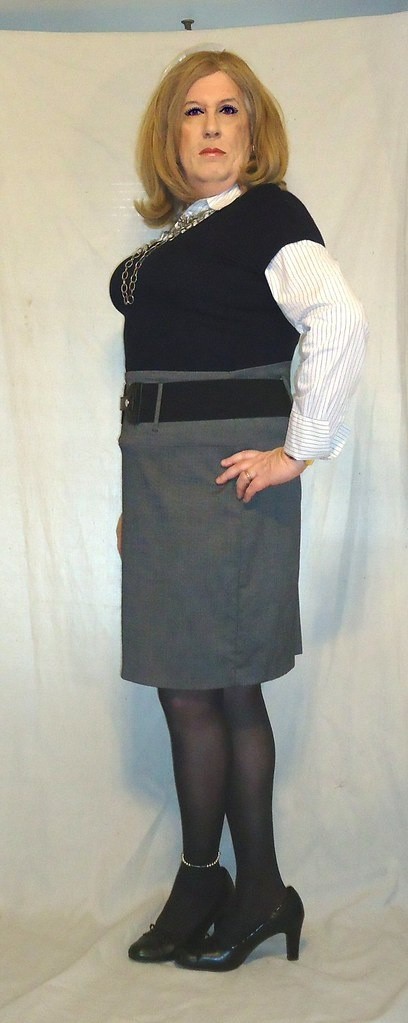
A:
[122,208,217,306]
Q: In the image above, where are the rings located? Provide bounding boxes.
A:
[245,470,252,482]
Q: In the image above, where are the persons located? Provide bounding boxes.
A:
[109,48,364,971]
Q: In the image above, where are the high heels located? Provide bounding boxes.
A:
[128,866,235,962]
[176,885,306,971]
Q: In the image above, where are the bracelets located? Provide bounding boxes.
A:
[284,453,314,465]
